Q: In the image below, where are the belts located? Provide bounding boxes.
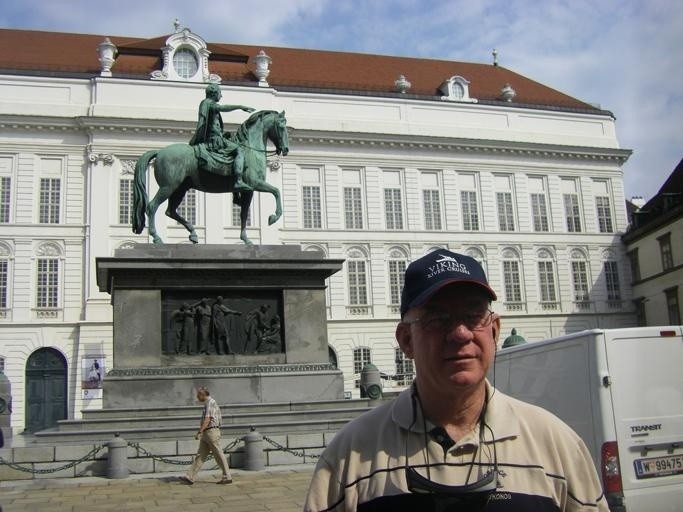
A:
[207,426,219,430]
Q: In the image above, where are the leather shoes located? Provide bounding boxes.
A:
[216,479,232,484]
[179,476,194,484]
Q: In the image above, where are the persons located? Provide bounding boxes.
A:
[189,81,256,191]
[304,247,613,512]
[179,387,232,485]
[175,295,283,356]
[90,360,101,381]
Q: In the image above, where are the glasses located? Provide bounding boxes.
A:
[405,419,498,494]
[410,306,494,335]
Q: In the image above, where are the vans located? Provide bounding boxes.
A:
[488,324,682,512]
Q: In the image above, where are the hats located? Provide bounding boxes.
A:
[400,248,497,318]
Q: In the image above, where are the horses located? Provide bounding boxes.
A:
[132,110,289,246]
